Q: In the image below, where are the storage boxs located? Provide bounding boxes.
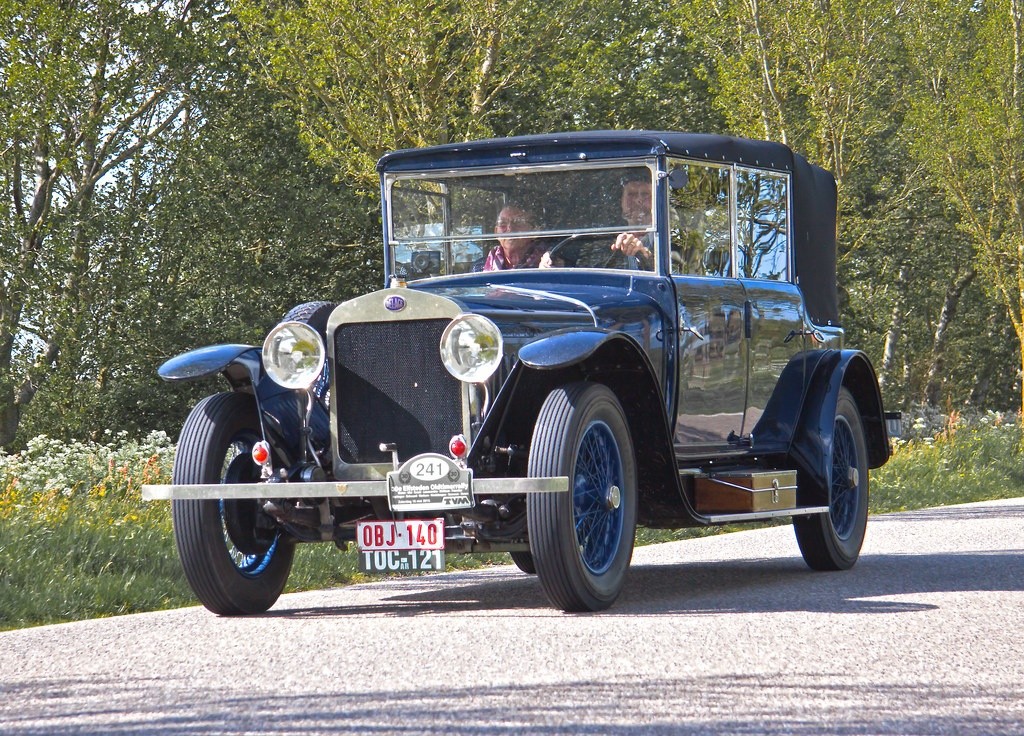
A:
[697,468,799,510]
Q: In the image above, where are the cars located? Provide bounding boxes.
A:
[140,129,900,613]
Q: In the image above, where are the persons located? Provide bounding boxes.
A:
[538,172,684,273]
[467,203,568,274]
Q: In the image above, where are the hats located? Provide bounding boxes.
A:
[620,166,653,184]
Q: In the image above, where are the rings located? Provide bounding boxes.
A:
[630,243,635,246]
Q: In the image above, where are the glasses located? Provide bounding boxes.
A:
[495,219,527,227]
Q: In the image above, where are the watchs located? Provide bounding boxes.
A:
[643,246,652,258]
